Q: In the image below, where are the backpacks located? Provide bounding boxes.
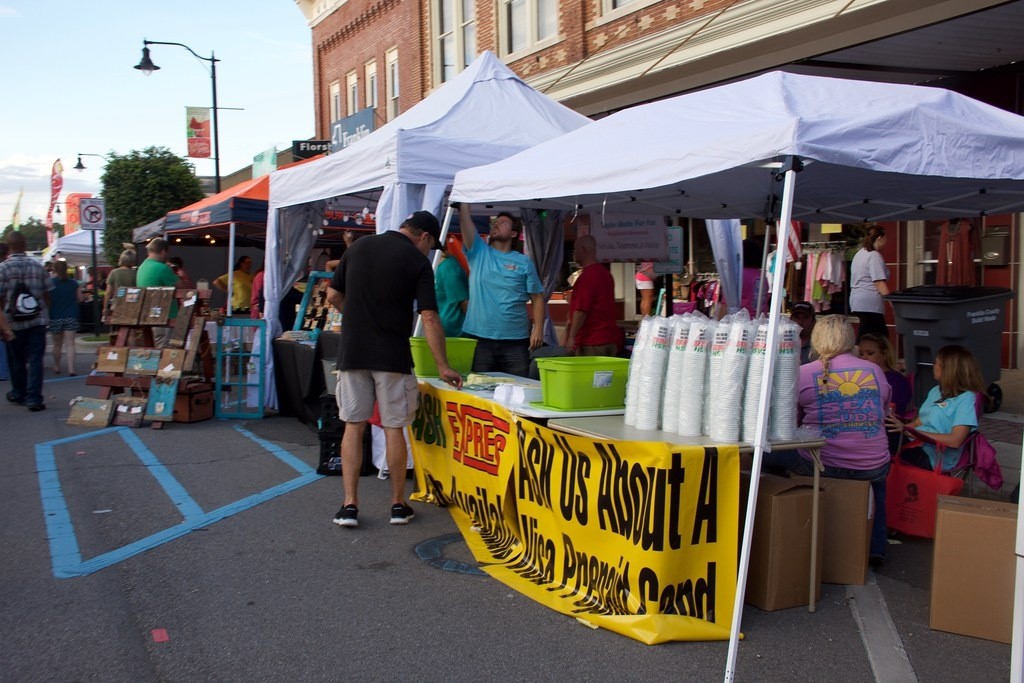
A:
[9,281,41,321]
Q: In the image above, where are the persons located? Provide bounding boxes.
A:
[1,183,986,545]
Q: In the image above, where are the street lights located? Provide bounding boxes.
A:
[133,37,245,194]
[54,201,100,336]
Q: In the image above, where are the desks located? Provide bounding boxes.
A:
[273,331,341,424]
[408,372,826,645]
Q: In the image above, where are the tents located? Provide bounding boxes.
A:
[42,48,1024,683]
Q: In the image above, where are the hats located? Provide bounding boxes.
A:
[790,301,816,318]
[399,210,445,252]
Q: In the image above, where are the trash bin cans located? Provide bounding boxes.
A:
[881,286,1012,413]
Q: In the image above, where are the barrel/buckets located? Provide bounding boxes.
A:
[322,357,339,396]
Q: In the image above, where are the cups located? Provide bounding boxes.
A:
[623,316,799,441]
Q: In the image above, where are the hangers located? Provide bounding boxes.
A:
[802,241,846,255]
[691,273,720,285]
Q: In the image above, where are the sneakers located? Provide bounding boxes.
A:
[389,502,416,524]
[333,505,358,526]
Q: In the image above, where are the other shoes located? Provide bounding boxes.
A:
[7,390,26,405]
[28,404,46,411]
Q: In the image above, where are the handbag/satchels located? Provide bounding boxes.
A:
[885,426,967,538]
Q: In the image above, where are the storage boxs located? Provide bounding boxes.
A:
[929,495,1017,644]
[409,337,478,376]
[535,356,630,408]
[740,468,870,611]
[174,383,215,424]
